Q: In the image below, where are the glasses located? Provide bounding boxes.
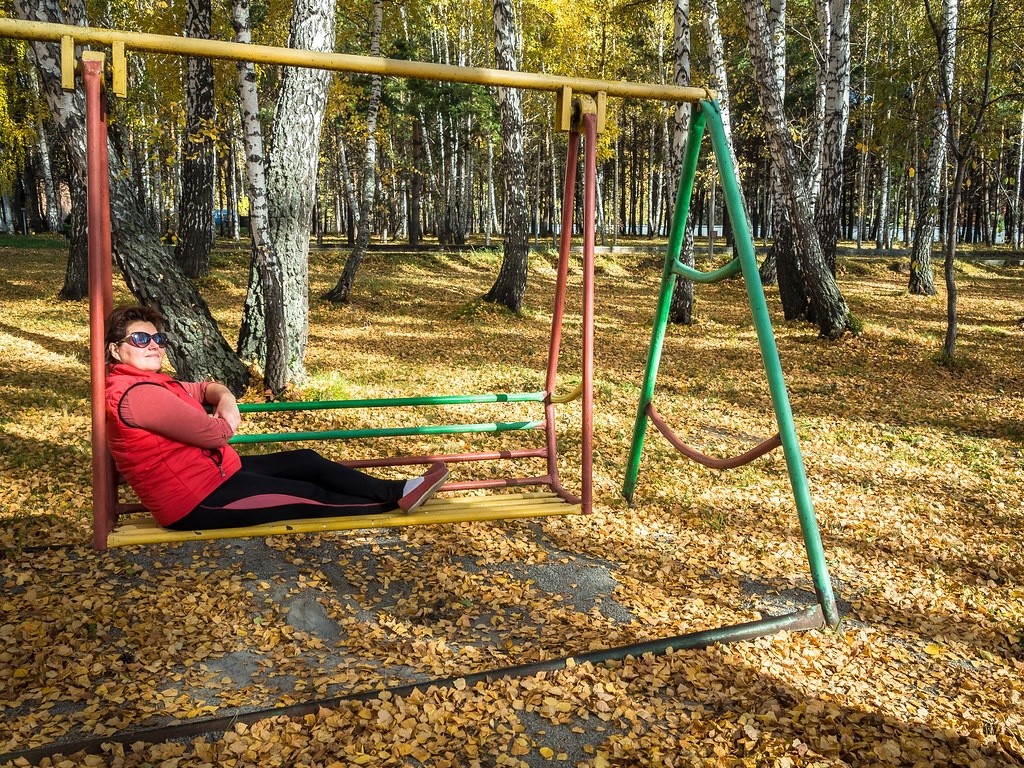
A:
[115,332,167,348]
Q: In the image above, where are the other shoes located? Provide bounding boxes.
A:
[422,460,444,507]
[398,467,450,515]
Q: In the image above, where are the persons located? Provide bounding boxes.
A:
[105,306,450,531]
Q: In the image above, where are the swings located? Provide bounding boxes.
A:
[78,51,601,555]
